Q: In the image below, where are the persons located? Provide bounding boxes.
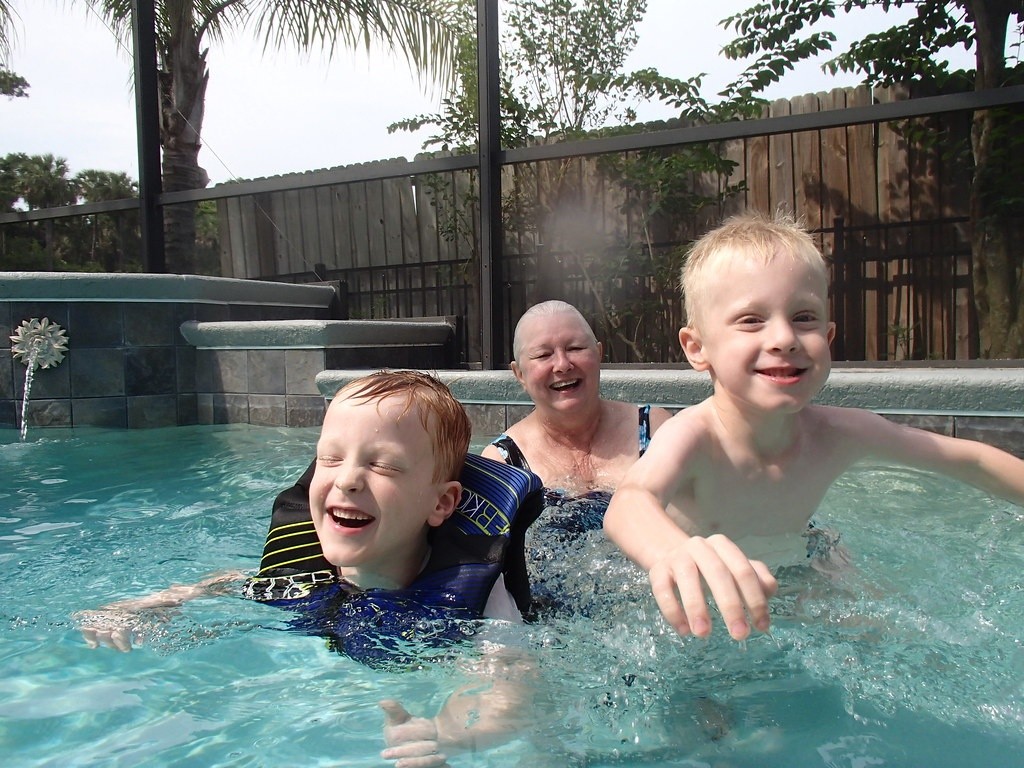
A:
[80,369,545,768]
[481,299,680,536]
[603,210,1023,640]
